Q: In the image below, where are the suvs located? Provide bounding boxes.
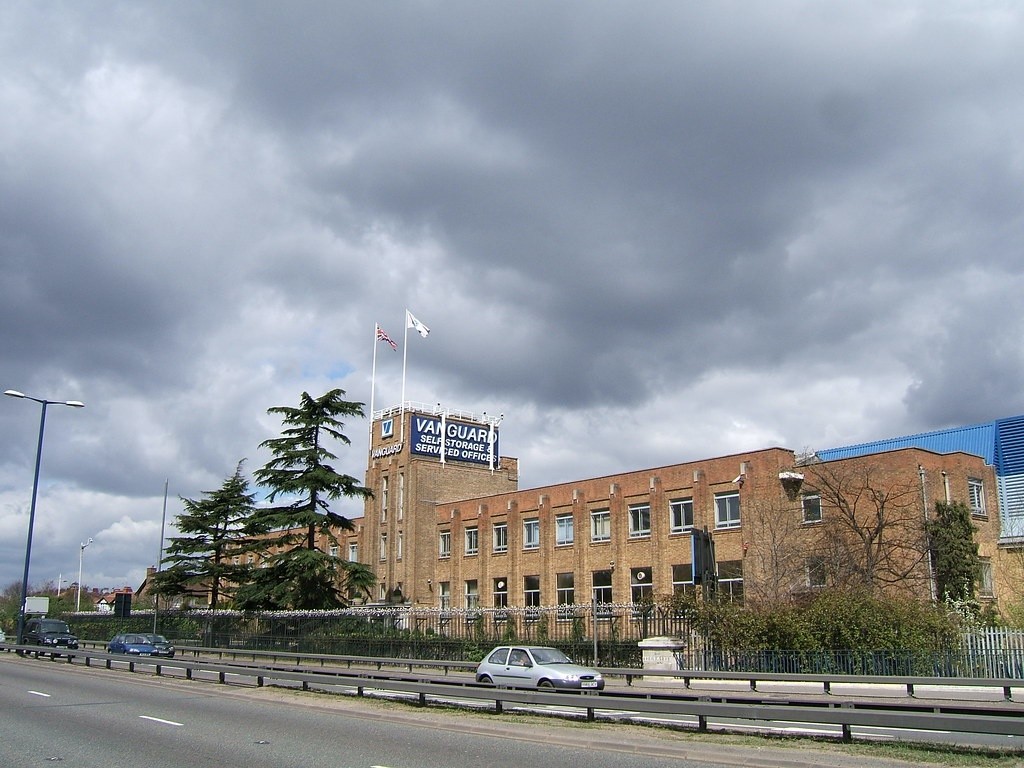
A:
[22,616,79,658]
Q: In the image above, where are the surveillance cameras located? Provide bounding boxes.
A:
[610,561,615,567]
[732,475,744,485]
[427,580,431,584]
[90,540,93,543]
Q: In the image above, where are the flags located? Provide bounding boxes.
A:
[407,310,430,338]
[377,327,397,352]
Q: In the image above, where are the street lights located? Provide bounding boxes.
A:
[4,389,85,653]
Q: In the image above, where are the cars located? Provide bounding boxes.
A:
[475,646,605,696]
[139,633,175,658]
[0,627,5,651]
[106,634,159,656]
